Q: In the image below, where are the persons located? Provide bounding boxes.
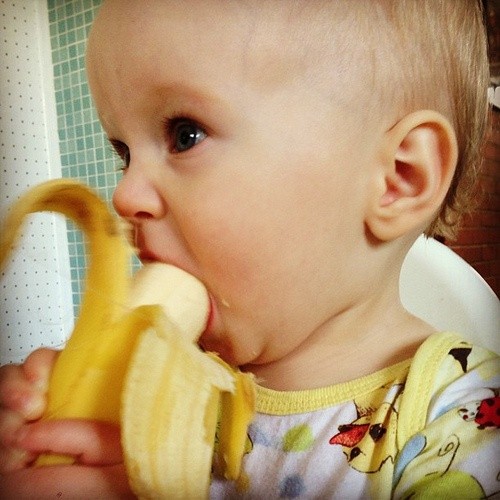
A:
[2,0,500,500]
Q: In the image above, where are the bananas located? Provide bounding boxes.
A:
[0,178,256,500]
